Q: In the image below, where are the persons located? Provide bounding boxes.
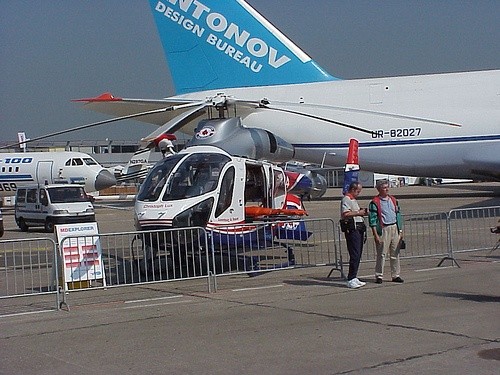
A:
[341,181,370,288]
[368,180,404,284]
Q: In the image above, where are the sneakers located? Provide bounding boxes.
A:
[347,278,366,289]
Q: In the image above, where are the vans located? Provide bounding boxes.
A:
[14,184,95,233]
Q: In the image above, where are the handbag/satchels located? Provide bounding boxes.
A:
[399,237,406,249]
[357,223,366,231]
[340,217,355,232]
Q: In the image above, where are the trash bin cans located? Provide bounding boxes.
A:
[398,176,405,187]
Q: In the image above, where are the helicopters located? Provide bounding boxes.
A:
[0,93,462,277]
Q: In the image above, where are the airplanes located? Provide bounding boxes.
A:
[0,151,117,237]
[72,0,500,184]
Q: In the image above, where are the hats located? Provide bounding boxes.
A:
[374,179,389,188]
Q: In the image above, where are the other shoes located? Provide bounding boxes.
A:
[376,278,382,284]
[392,277,404,283]
